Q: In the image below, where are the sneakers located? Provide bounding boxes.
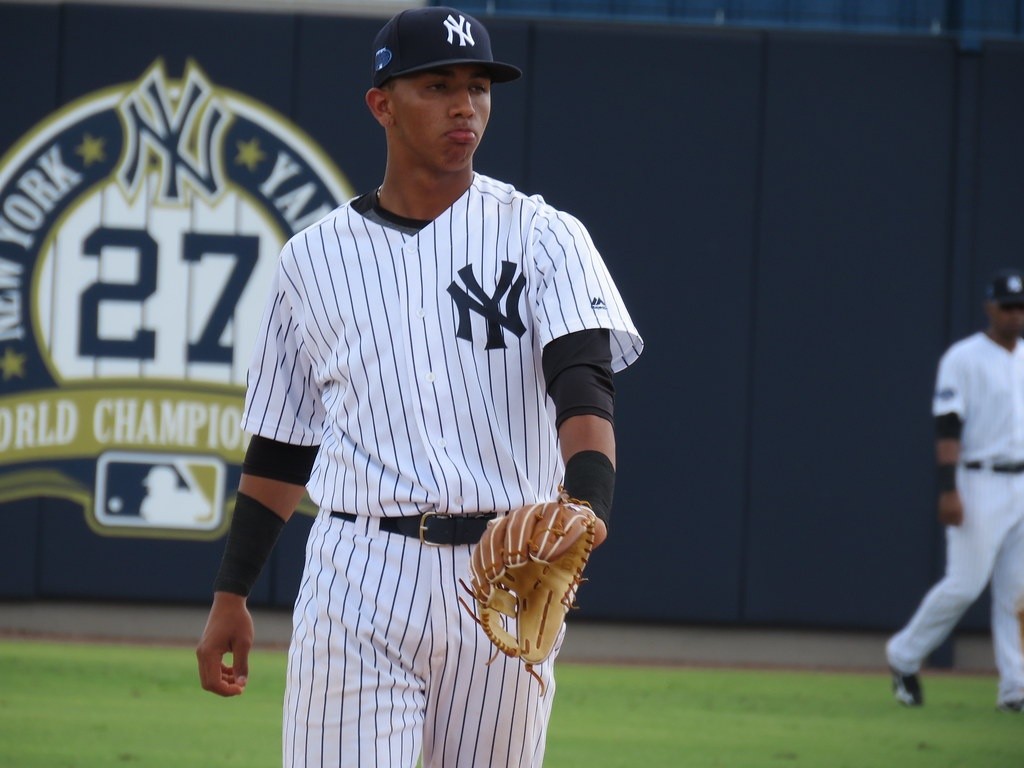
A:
[891,664,922,706]
[998,701,1023,711]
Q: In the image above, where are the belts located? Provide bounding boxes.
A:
[328,509,495,546]
[965,457,1022,476]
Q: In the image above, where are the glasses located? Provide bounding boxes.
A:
[996,299,1024,311]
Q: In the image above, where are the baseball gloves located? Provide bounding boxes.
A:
[470,498,610,669]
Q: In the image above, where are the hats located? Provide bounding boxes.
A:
[371,6,522,92]
[988,272,1024,305]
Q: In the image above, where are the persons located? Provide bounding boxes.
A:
[884,278,1024,712]
[195,6,645,768]
